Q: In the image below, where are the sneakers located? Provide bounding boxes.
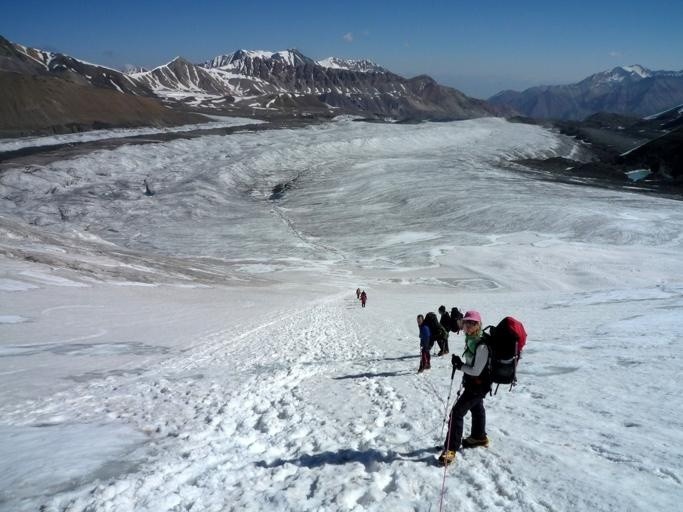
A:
[462,437,489,447]
[440,448,455,463]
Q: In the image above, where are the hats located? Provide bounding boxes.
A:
[463,311,480,322]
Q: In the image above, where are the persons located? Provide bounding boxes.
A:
[359,294,367,308]
[416,314,432,373]
[356,288,361,299]
[360,290,366,295]
[436,310,490,463]
[438,304,450,356]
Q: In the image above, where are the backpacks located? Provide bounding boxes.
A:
[474,317,527,384]
[425,312,440,341]
[450,308,463,332]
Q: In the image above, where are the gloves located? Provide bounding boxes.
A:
[452,355,464,370]
